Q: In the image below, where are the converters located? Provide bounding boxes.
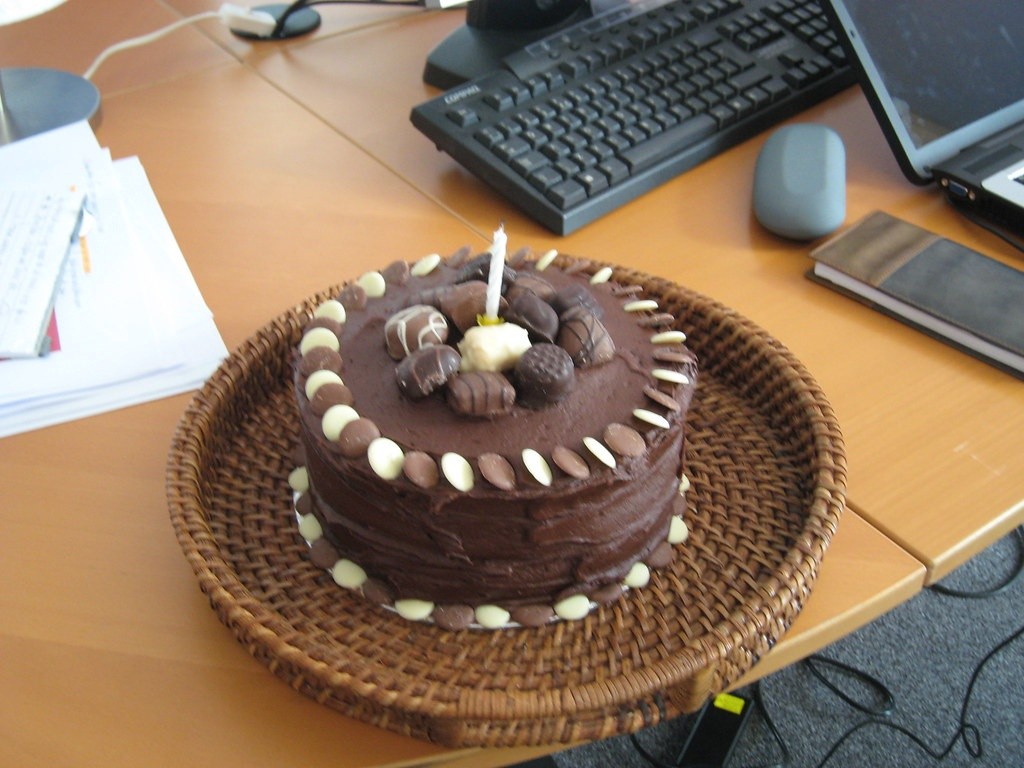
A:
[675,690,756,768]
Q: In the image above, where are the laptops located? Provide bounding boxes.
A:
[819,0,1024,241]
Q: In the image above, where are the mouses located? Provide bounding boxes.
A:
[753,122,846,241]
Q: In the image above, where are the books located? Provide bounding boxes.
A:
[0,151,90,360]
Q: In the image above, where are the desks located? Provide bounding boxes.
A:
[0,0,1024,768]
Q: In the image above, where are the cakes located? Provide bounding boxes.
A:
[287,244,699,629]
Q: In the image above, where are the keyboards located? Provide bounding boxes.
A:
[407,0,861,237]
